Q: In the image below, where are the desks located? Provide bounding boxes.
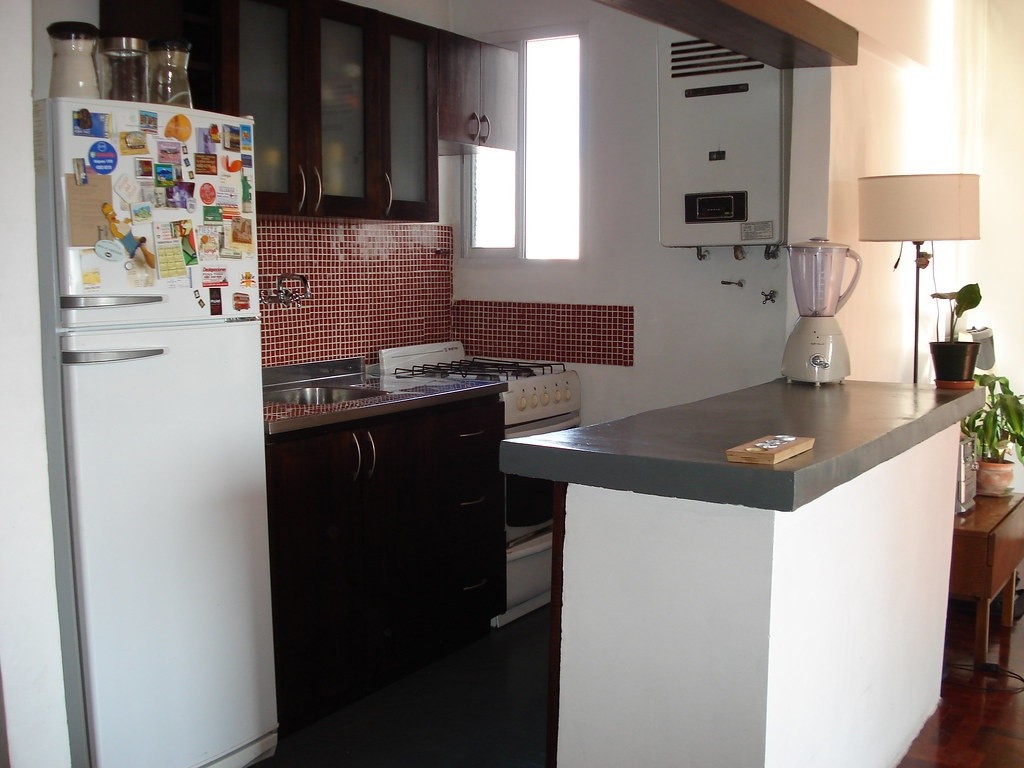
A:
[949,493,1024,670]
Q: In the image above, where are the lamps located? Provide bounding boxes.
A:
[858,174,980,383]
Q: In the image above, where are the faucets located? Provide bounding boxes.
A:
[277,273,310,300]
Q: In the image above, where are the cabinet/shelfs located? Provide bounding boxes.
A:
[99,0,520,224]
[265,394,506,725]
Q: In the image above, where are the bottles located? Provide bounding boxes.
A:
[147,38,193,108]
[96,35,146,102]
[46,21,100,101]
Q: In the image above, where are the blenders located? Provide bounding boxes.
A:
[781,236,863,388]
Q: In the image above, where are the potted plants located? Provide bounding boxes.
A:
[929,283,982,392]
[960,374,1024,497]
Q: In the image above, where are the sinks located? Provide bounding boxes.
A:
[264,386,389,405]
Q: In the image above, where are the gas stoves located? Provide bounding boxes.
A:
[387,343,575,387]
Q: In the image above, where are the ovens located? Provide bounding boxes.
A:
[503,408,581,611]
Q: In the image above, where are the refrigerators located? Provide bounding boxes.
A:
[31,99,279,768]
